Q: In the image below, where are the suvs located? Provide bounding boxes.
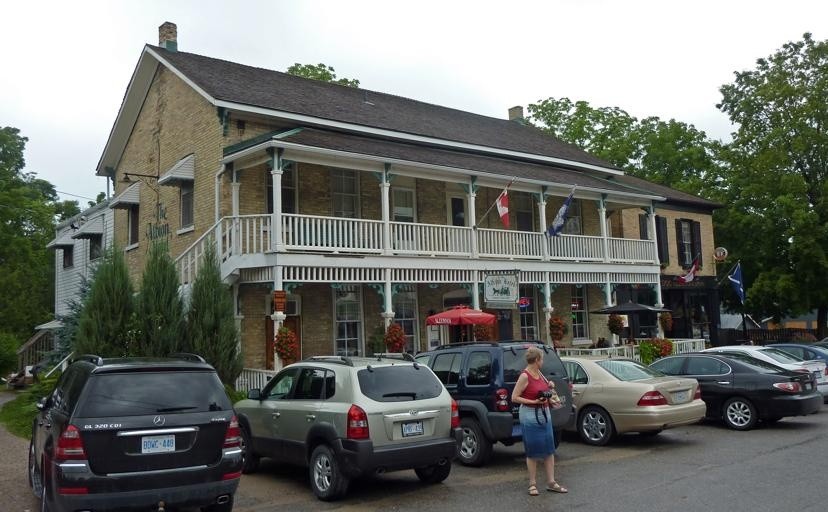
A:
[24,348,242,511]
[233,350,459,505]
[413,338,575,467]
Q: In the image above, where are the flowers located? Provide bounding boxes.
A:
[273,325,299,361]
[658,310,674,332]
[381,319,405,354]
[548,315,568,349]
[607,314,625,336]
[473,322,491,340]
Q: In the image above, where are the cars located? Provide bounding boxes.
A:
[700,345,827,401]
[811,337,827,349]
[764,344,827,367]
[558,354,706,448]
[646,354,823,432]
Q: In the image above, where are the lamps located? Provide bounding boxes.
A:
[114,169,161,195]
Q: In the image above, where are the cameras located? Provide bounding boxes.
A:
[538,390,552,399]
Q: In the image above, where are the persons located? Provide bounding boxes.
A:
[510,346,569,495]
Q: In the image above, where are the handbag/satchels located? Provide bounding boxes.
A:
[548,388,562,410]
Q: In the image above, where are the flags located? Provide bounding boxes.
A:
[678,256,699,284]
[728,263,744,306]
[496,180,512,230]
[548,188,574,237]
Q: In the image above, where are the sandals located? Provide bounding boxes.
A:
[546,480,567,493]
[528,484,539,495]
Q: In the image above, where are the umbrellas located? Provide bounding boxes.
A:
[425,303,495,345]
[589,298,673,345]
